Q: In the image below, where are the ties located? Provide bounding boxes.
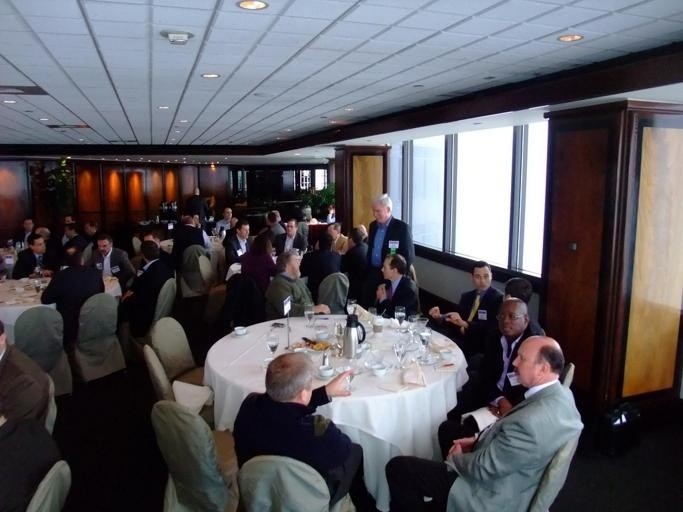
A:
[505,343,512,358]
[387,284,392,298]
[467,295,479,321]
[36,256,42,265]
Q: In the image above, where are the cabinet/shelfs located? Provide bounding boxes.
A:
[539,100,683,416]
[334,145,391,237]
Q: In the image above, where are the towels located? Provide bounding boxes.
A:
[461,407,499,431]
[7,297,35,306]
[172,379,215,415]
[388,317,410,331]
[403,367,427,387]
[436,361,460,372]
[378,374,405,392]
[428,339,457,354]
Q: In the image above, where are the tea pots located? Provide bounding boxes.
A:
[343,314,366,359]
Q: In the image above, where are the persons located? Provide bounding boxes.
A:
[36,227,62,252]
[438,297,545,462]
[504,277,534,305]
[233,352,381,512]
[376,254,420,321]
[325,204,336,223]
[224,220,249,265]
[219,217,238,243]
[0,321,48,422]
[0,412,59,512]
[275,219,307,254]
[298,204,318,225]
[216,208,232,231]
[340,224,370,305]
[139,232,169,268]
[256,210,285,243]
[84,220,103,243]
[429,260,504,354]
[314,223,349,255]
[41,242,104,351]
[241,236,276,281]
[300,233,342,299]
[366,194,415,279]
[118,240,174,336]
[62,215,76,231]
[176,216,211,254]
[266,251,331,318]
[327,223,348,255]
[63,223,89,260]
[92,235,134,278]
[188,187,205,215]
[385,336,584,512]
[12,233,73,280]
[12,218,37,248]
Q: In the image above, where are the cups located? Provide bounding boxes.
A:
[266,331,278,359]
[407,315,432,363]
[304,306,315,329]
[316,329,329,340]
[394,343,407,373]
[395,306,406,333]
[235,326,246,336]
[319,365,334,377]
[343,361,357,393]
[440,349,453,361]
[367,307,377,330]
[372,364,387,378]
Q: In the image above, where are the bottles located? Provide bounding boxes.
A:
[323,352,328,367]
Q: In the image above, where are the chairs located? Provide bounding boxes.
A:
[131,277,177,357]
[26,459,71,512]
[528,431,579,512]
[143,344,215,427]
[45,370,58,435]
[151,400,238,512]
[183,243,227,319]
[64,293,127,384]
[318,272,347,314]
[147,316,204,385]
[237,455,331,512]
[13,305,73,397]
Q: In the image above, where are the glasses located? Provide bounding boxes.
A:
[496,313,525,321]
[474,274,490,279]
